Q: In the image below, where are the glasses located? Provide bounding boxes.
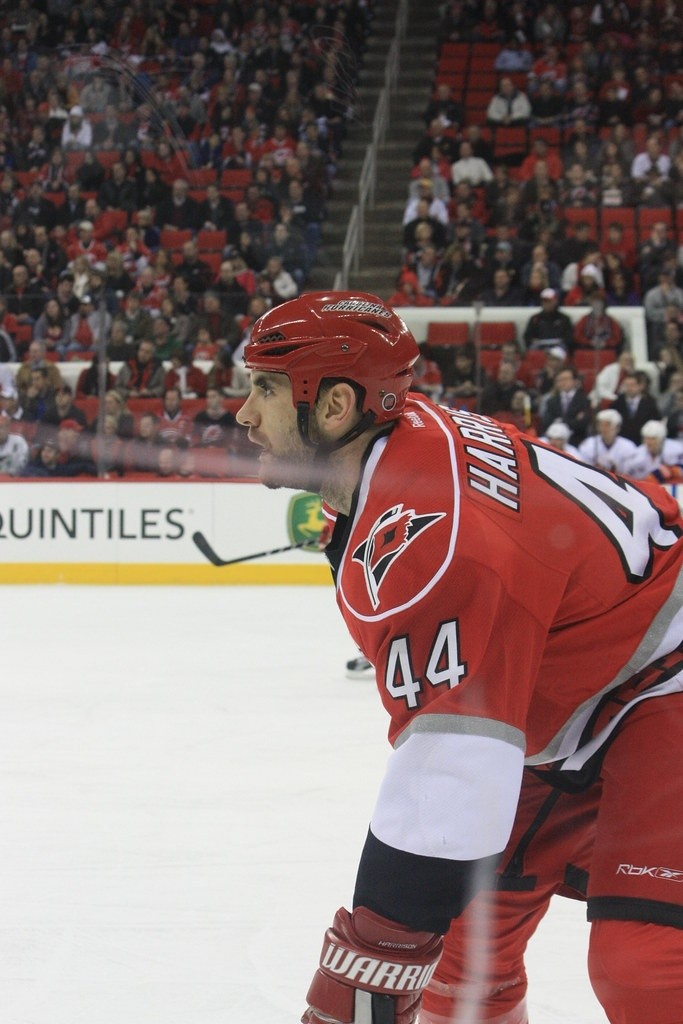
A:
[548,355,558,361]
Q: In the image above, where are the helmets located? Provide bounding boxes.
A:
[243,291,423,430]
[546,422,571,439]
[641,421,666,439]
[596,409,621,425]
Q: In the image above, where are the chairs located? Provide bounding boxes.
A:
[0,0,681,489]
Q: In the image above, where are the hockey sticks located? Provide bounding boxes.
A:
[191,531,347,569]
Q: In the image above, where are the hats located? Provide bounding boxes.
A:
[79,222,93,231]
[71,107,82,116]
[541,288,555,301]
[549,346,565,361]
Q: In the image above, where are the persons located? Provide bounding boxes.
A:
[1,0,388,478]
[402,1,682,484]
[237,289,682,1023]
